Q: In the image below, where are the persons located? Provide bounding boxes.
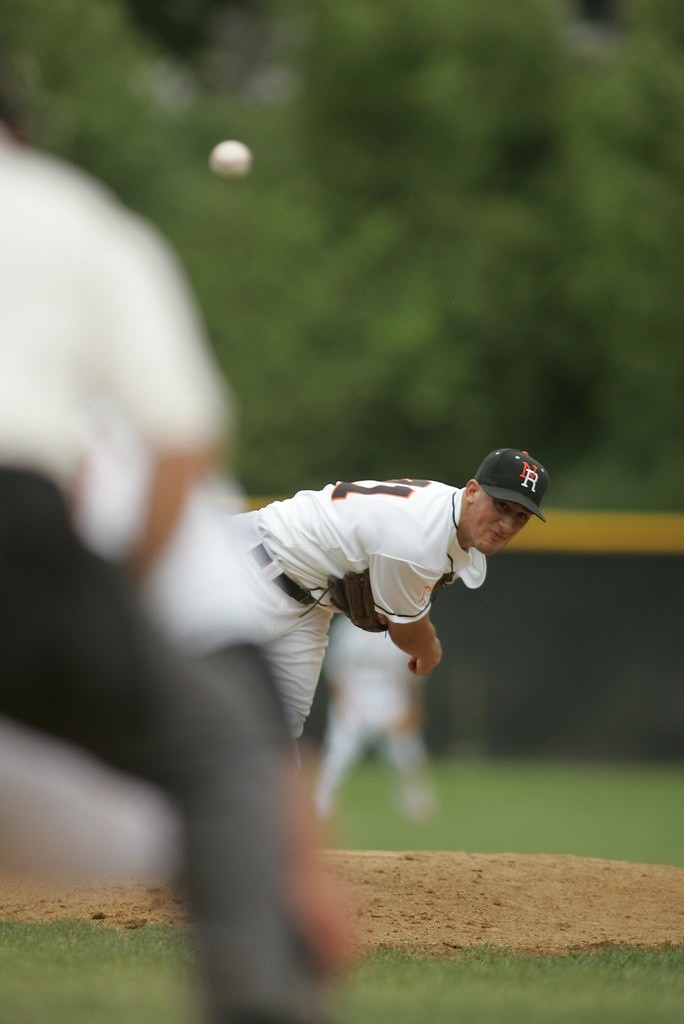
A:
[313,615,437,821]
[229,446,549,769]
[0,107,353,1024]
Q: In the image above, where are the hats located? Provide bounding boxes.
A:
[474,449,548,523]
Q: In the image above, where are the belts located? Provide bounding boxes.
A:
[252,543,316,605]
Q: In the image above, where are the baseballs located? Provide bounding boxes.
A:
[208,137,254,182]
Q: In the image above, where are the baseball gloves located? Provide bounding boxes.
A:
[327,566,389,633]
